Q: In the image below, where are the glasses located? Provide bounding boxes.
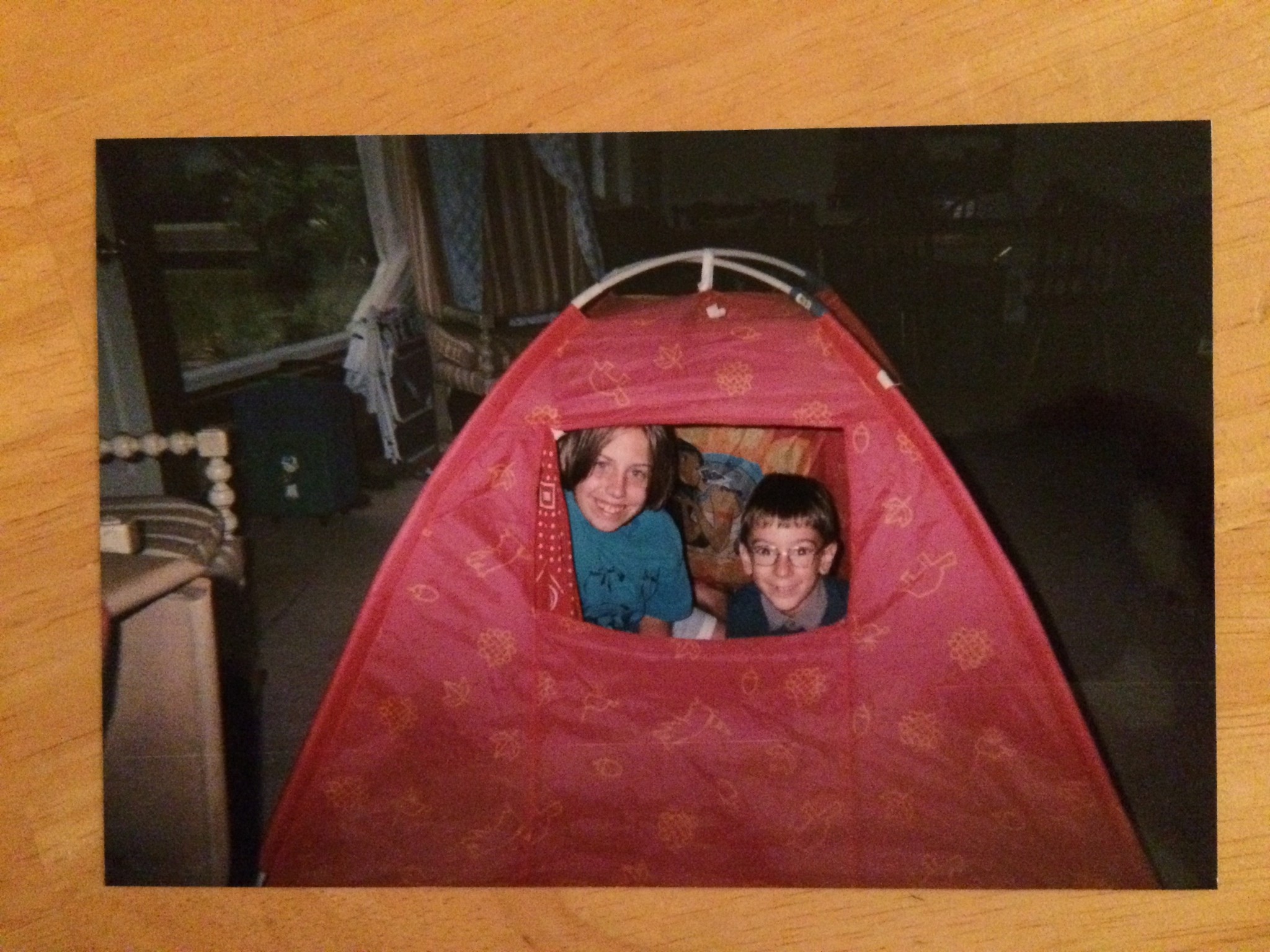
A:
[746,538,829,568]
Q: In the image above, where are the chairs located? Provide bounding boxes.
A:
[410,135,584,448]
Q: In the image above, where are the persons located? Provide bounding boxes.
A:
[553,419,698,642]
[721,471,849,640]
